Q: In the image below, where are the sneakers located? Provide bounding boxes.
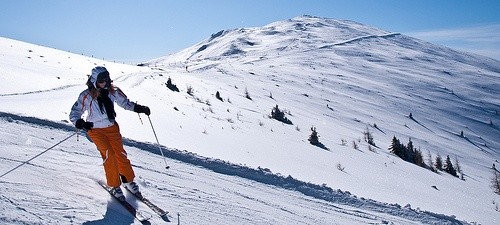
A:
[111,186,125,200]
[123,179,139,194]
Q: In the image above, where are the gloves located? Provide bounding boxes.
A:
[75,119,93,132]
[133,104,150,115]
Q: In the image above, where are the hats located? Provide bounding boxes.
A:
[90,66,108,89]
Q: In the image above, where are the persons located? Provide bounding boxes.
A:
[69,66,151,202]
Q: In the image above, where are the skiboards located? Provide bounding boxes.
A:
[97,174,170,222]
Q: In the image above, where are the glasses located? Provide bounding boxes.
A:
[97,78,109,84]
